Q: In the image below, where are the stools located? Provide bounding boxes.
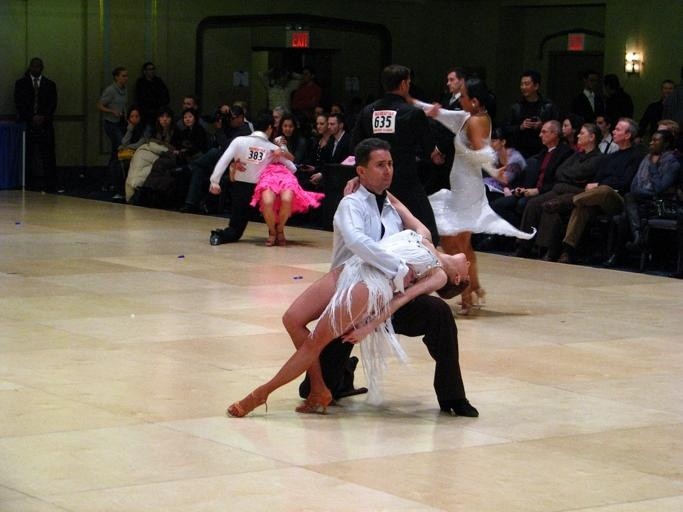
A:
[498,185,681,278]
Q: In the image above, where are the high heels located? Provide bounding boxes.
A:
[295,388,332,415]
[456,288,486,316]
[227,388,268,417]
[265,228,286,246]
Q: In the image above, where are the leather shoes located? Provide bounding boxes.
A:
[556,250,577,263]
[603,251,625,266]
[636,249,652,273]
[542,248,555,260]
[347,356,359,373]
[627,231,646,247]
[543,198,560,214]
[210,229,221,245]
[514,246,529,257]
[478,236,503,251]
[439,398,479,416]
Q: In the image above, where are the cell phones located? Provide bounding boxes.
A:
[531,116,537,122]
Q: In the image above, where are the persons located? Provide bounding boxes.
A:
[15,58,58,187]
[299,138,479,417]
[228,176,471,420]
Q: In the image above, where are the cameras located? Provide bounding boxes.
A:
[215,110,232,124]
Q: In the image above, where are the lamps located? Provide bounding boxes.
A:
[623,51,645,76]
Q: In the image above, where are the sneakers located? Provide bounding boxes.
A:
[178,202,200,213]
[165,167,180,180]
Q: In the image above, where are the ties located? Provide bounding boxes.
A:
[33,79,40,113]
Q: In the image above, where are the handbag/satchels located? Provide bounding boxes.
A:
[657,199,680,218]
[118,148,136,160]
